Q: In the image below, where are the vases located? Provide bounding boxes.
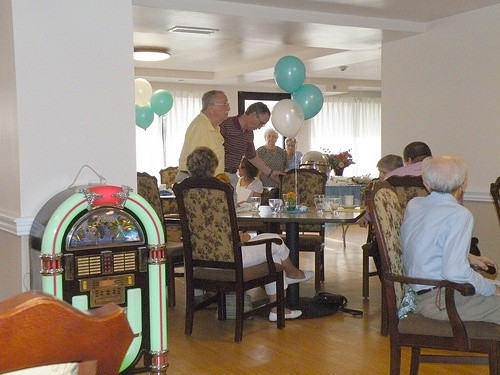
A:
[333,168,343,177]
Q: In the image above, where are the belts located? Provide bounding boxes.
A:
[224,168,238,174]
[416,287,437,295]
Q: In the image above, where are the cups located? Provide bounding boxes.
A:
[314,194,325,216]
[329,198,341,215]
[268,199,282,214]
[251,197,261,211]
[344,195,353,207]
[259,205,271,218]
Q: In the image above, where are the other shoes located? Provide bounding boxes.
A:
[269,310,302,321]
[285,269,314,284]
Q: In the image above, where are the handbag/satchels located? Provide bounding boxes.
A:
[216,294,253,320]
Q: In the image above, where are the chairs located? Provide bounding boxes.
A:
[137,168,190,306]
[363,175,500,375]
[273,170,327,289]
[172,176,286,342]
[0,290,135,375]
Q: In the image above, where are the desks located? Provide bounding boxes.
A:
[165,207,367,307]
[324,183,365,203]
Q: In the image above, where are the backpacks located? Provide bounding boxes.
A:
[300,292,348,318]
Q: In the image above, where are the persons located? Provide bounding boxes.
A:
[234,158,264,211]
[175,91,230,184]
[187,147,314,321]
[220,102,286,209]
[377,155,403,181]
[283,137,302,171]
[399,154,500,324]
[256,129,288,189]
[360,142,432,286]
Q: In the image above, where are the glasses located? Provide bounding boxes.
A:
[210,100,230,106]
[253,111,266,127]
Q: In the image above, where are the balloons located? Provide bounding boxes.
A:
[134,79,153,107]
[136,107,154,130]
[150,89,174,116]
[272,99,304,138]
[294,85,323,120]
[274,56,306,93]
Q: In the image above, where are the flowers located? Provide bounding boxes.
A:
[324,150,353,171]
